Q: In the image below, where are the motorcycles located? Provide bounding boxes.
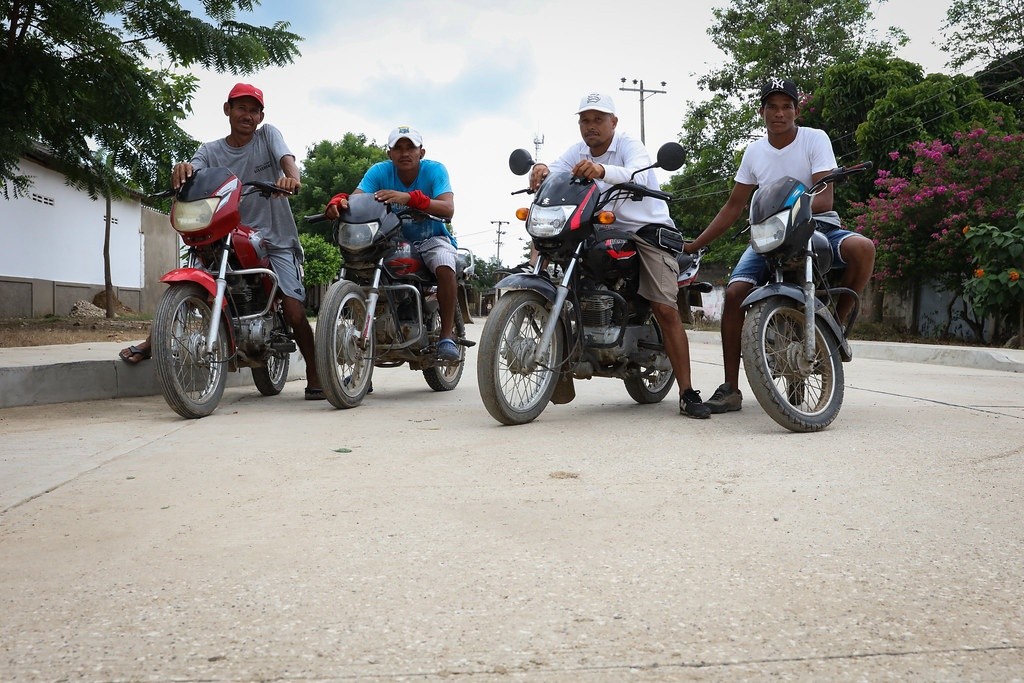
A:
[303,193,480,409]
[147,166,305,419]
[730,161,874,433]
[477,142,713,425]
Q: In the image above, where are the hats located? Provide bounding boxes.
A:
[760,79,798,106]
[387,126,423,153]
[229,83,264,112]
[575,94,616,117]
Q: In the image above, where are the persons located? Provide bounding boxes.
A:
[324,126,460,393]
[529,92,711,419]
[684,76,876,415]
[119,84,326,400]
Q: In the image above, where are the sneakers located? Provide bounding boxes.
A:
[703,385,742,413]
[495,261,551,282]
[679,388,712,419]
[839,326,853,362]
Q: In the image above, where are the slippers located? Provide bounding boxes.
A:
[119,346,152,364]
[437,339,460,361]
[305,386,325,400]
[343,375,373,392]
